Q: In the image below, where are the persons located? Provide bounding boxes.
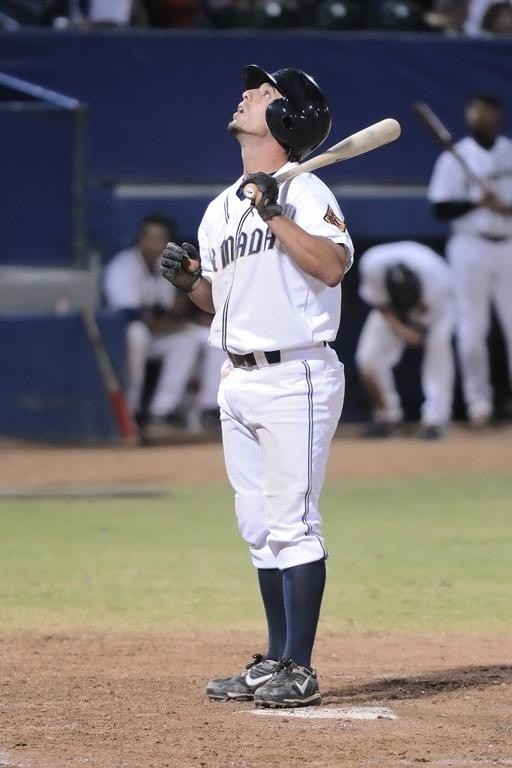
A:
[424,92,512,429]
[352,239,459,440]
[157,59,361,710]
[101,213,201,438]
[182,301,228,436]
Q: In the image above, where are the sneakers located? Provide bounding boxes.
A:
[206,654,321,708]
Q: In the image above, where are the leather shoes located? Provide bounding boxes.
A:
[236,172,283,222]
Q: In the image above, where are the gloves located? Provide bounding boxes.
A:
[159,241,203,294]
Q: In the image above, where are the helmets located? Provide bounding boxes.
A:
[242,63,332,163]
[386,264,420,308]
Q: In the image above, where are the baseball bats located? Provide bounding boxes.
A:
[243,118,401,198]
[412,103,491,195]
[84,311,138,443]
